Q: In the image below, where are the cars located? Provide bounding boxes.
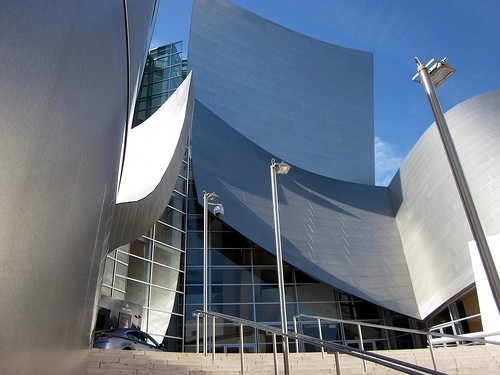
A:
[92,328,169,352]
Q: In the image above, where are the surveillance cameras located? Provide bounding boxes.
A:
[213,205,224,218]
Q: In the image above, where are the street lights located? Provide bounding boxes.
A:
[203,190,224,358]
[410,55,500,312]
[270,157,293,375]
